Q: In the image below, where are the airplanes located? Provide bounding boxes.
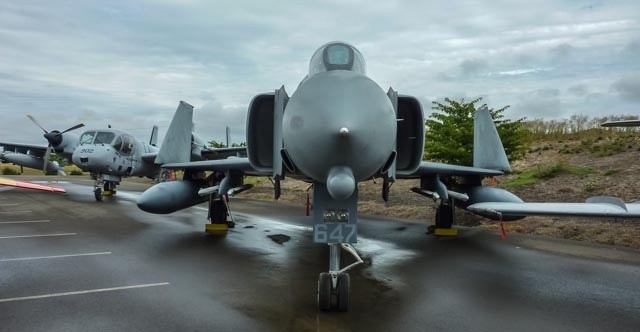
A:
[137,40,529,314]
[0,115,247,200]
